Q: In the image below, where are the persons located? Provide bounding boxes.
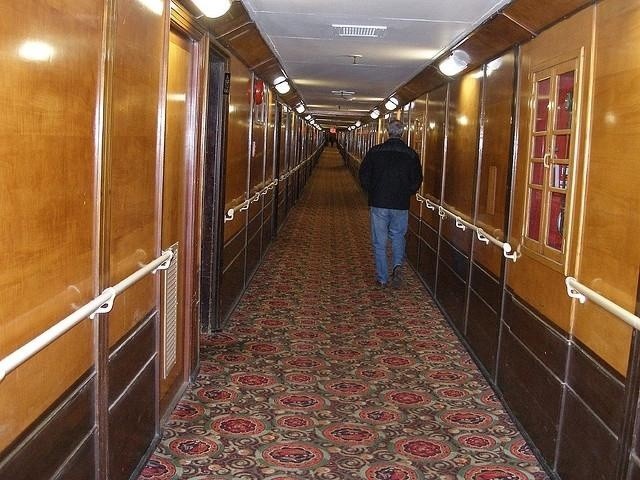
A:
[358,120,424,290]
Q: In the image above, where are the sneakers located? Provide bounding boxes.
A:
[376,265,404,287]
